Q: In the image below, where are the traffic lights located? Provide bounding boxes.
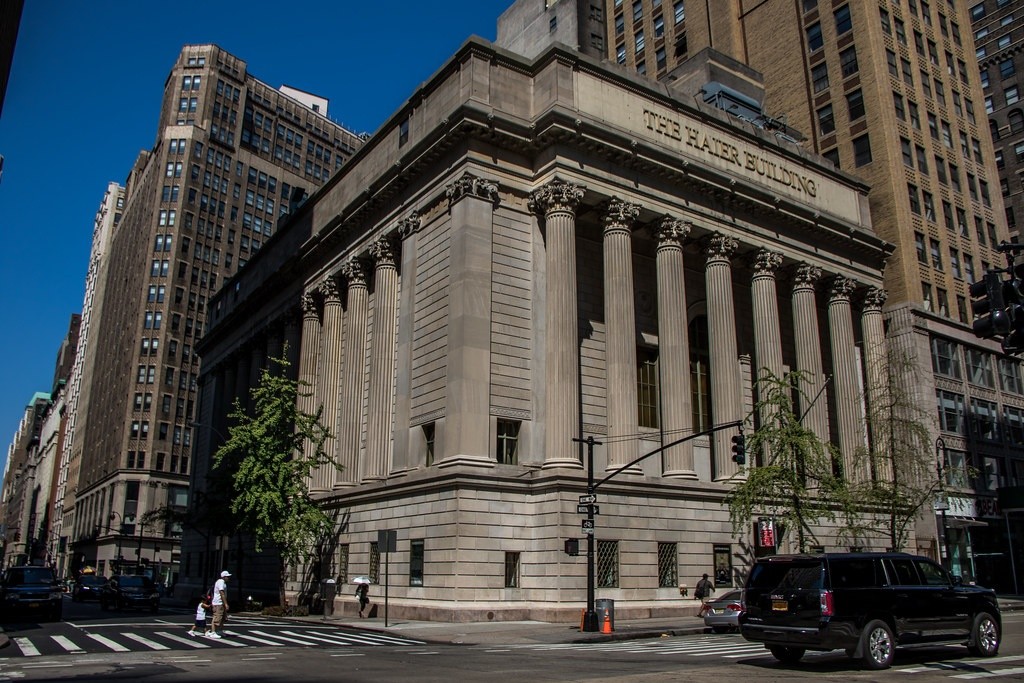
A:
[968,273,1008,339]
[997,280,1024,356]
[731,435,745,464]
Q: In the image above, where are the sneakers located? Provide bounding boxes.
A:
[187,630,196,637]
[210,632,221,639]
[205,631,211,637]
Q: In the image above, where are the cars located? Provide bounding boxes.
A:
[101,575,160,611]
[199,585,236,614]
[73,575,110,601]
[704,591,741,631]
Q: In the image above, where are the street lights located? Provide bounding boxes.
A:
[936,437,951,570]
[109,511,136,574]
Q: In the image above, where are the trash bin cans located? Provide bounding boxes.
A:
[321,578,336,600]
[595,597,616,636]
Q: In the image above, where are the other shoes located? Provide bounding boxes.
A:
[697,614,702,617]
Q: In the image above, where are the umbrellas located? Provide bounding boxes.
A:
[351,577,375,584]
[82,566,98,575]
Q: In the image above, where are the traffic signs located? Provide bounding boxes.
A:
[576,506,600,515]
[579,494,597,504]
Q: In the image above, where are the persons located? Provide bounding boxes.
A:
[157,573,179,598]
[355,583,369,618]
[694,573,715,618]
[187,571,232,639]
[70,575,76,592]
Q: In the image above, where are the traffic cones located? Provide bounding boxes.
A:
[601,608,612,633]
[580,609,586,632]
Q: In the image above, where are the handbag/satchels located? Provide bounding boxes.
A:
[694,587,705,599]
[366,598,369,603]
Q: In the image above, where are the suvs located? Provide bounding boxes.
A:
[739,549,1002,667]
[0,566,62,620]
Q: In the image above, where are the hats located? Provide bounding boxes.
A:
[221,571,232,577]
[703,574,708,578]
